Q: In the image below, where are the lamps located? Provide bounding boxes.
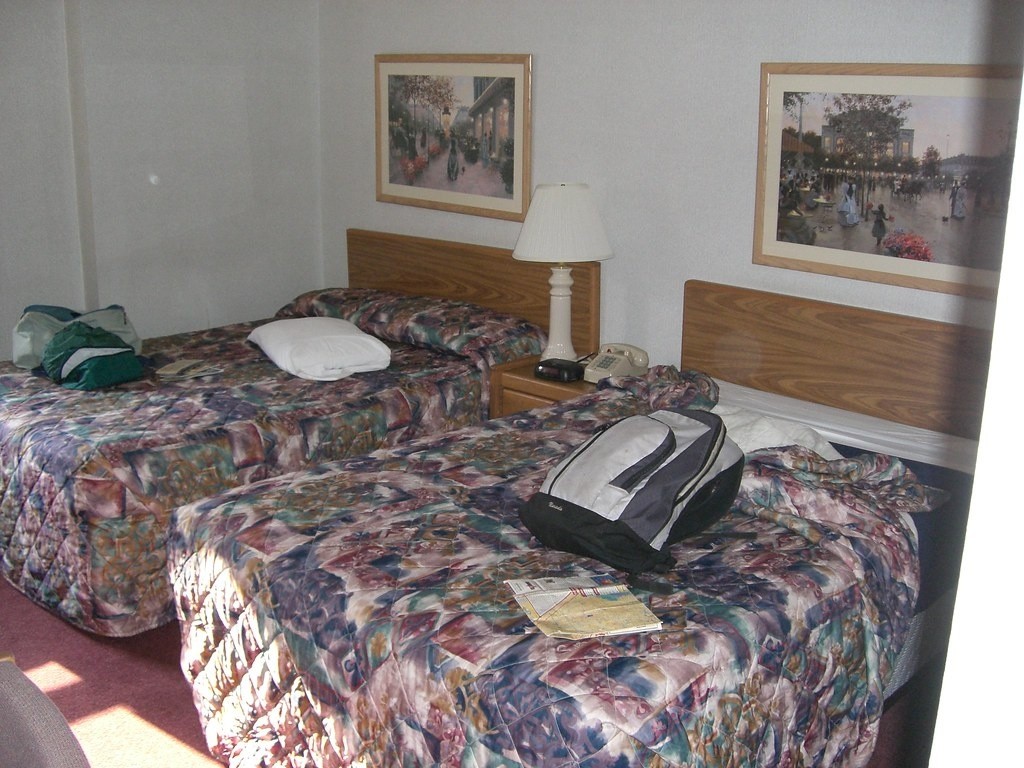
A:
[512,184,615,360]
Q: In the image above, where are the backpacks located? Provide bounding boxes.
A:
[517,409,744,577]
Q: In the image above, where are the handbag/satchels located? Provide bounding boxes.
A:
[12,305,145,390]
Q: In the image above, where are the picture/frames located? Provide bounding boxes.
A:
[375,54,532,222]
[752,63,1024,301]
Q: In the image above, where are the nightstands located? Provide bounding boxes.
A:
[490,352,597,419]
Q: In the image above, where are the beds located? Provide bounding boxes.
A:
[0,227,601,637]
[167,279,994,768]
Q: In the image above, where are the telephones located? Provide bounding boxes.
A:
[583,343,649,385]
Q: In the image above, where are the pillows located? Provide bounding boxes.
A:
[247,318,391,381]
[710,405,846,461]
